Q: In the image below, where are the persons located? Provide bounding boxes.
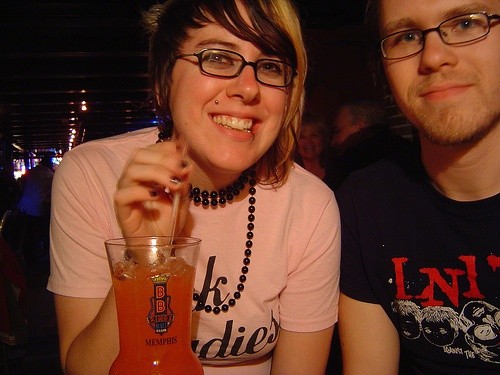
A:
[0,155,61,375]
[290,94,420,190]
[336,0,500,375]
[45,0,341,375]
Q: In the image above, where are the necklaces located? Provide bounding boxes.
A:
[156,118,256,314]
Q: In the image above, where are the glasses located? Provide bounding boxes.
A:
[378,12,499,60]
[173,49,291,89]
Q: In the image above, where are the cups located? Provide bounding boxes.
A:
[104,235,205,375]
